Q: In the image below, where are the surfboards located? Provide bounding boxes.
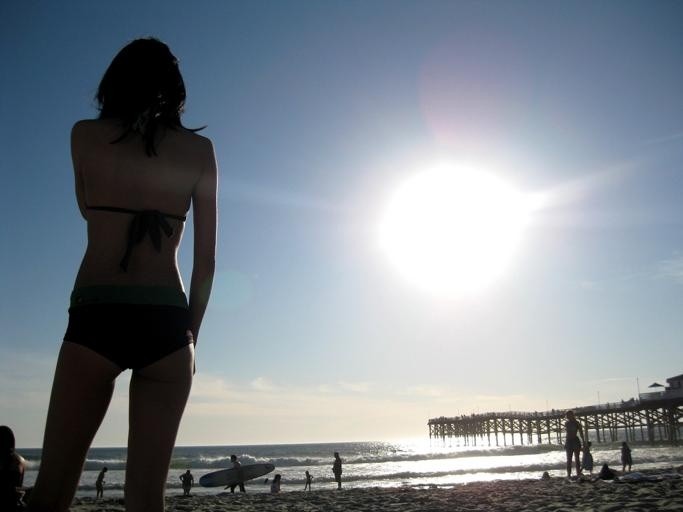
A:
[199,464,275,487]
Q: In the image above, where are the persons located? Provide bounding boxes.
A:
[620,442,632,472]
[30,39,217,512]
[271,474,281,493]
[231,455,246,493]
[564,411,584,476]
[96,466,108,498]
[0,426,26,512]
[179,470,194,495]
[303,471,313,491]
[331,452,342,490]
[580,441,593,475]
